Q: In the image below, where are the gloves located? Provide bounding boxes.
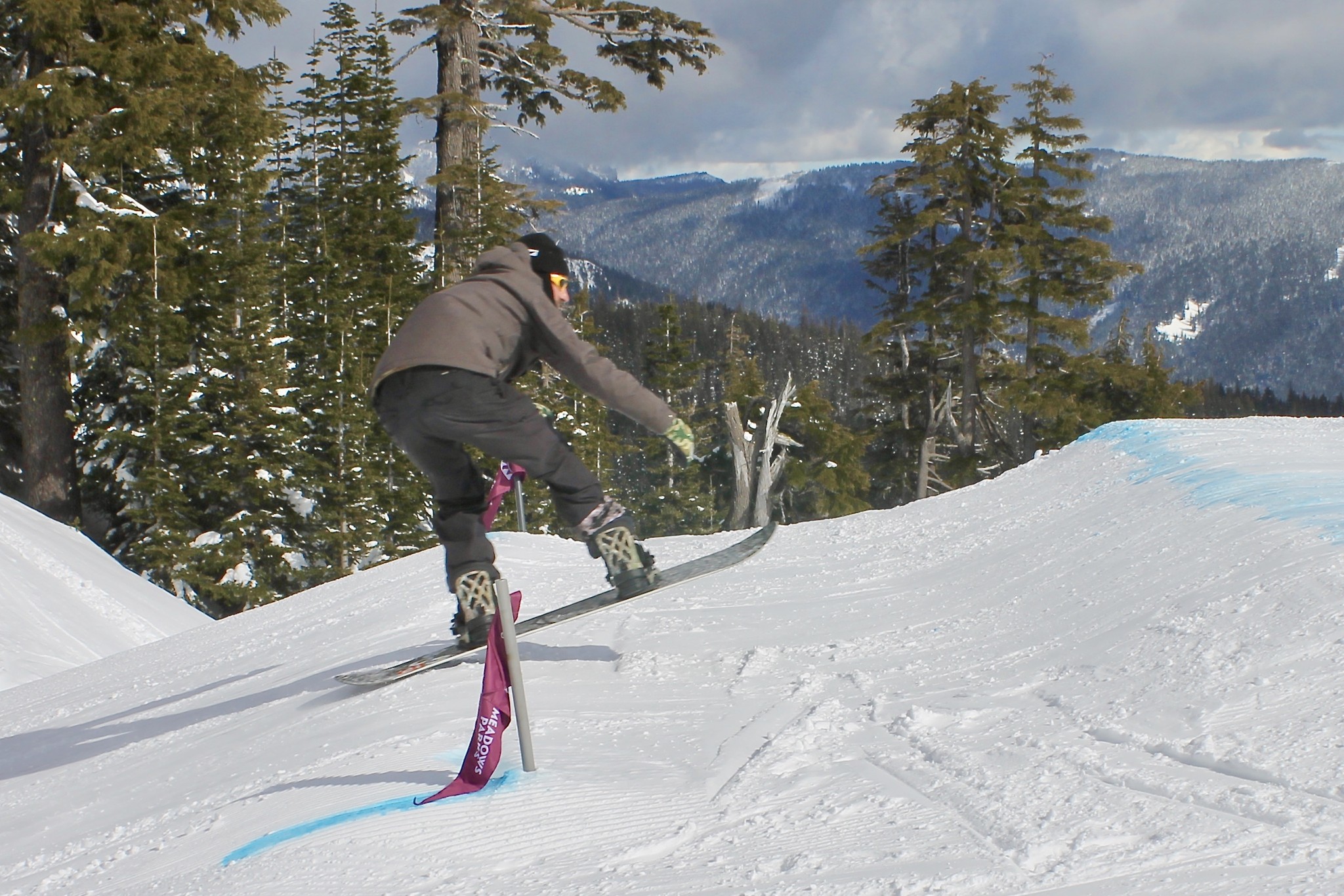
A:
[532,403,555,425]
[657,418,695,467]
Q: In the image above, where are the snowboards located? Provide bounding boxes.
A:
[333,520,780,687]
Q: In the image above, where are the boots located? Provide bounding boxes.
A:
[586,515,660,601]
[449,562,508,643]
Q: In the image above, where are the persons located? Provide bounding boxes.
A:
[367,230,697,648]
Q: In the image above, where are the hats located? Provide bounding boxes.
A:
[513,233,571,278]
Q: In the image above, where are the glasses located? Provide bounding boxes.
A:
[550,273,569,290]
